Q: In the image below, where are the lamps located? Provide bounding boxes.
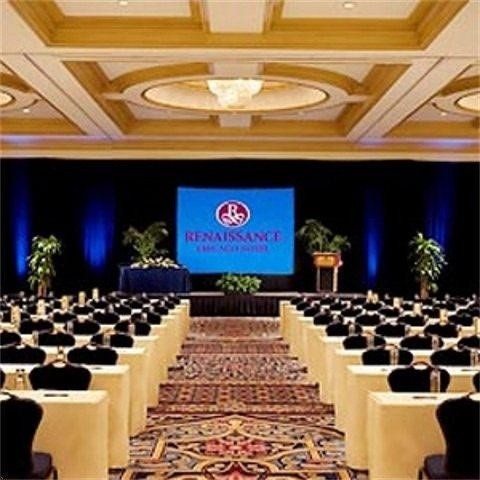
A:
[206,79,263,110]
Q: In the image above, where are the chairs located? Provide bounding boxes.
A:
[1,292,195,480]
[277,286,480,480]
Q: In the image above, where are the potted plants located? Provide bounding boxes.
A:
[293,217,354,270]
[214,271,264,317]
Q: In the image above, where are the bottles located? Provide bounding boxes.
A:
[0,287,170,392]
[302,295,480,397]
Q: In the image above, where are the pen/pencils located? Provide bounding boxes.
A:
[461,369,480,371]
[414,396,436,398]
[92,367,102,369]
[44,394,67,397]
